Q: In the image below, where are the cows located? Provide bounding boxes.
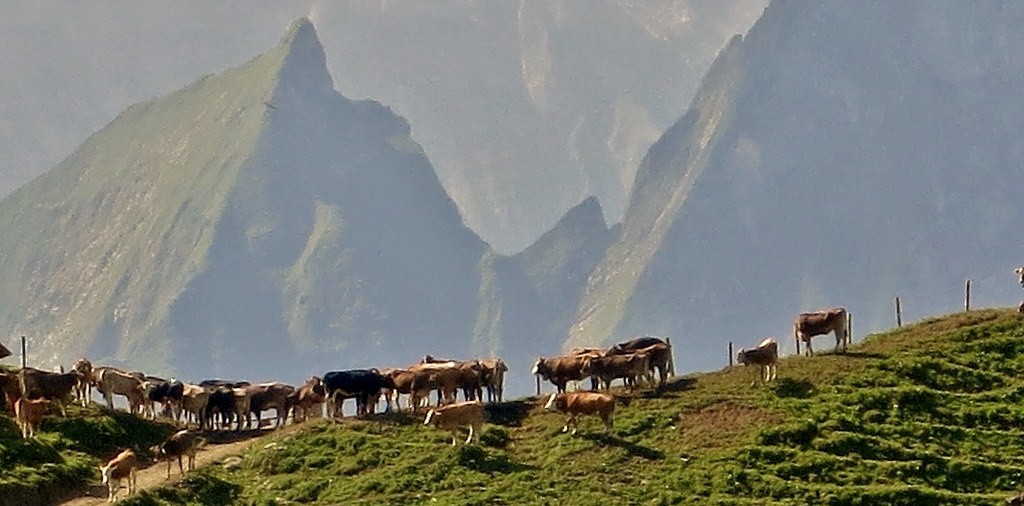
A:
[0,356,508,447]
[532,337,674,438]
[98,448,138,503]
[148,430,198,480]
[735,338,781,382]
[794,307,852,357]
